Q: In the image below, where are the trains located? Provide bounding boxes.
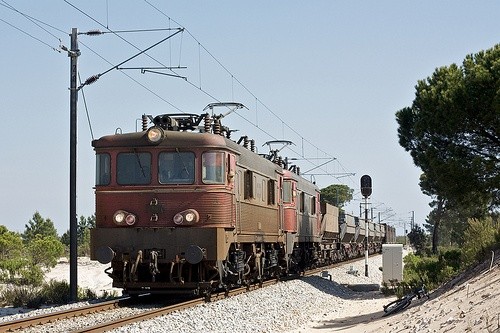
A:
[91,113,396,295]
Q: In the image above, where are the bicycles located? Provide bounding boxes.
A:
[383,283,429,317]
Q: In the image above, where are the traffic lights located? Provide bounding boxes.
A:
[360,175,372,197]
